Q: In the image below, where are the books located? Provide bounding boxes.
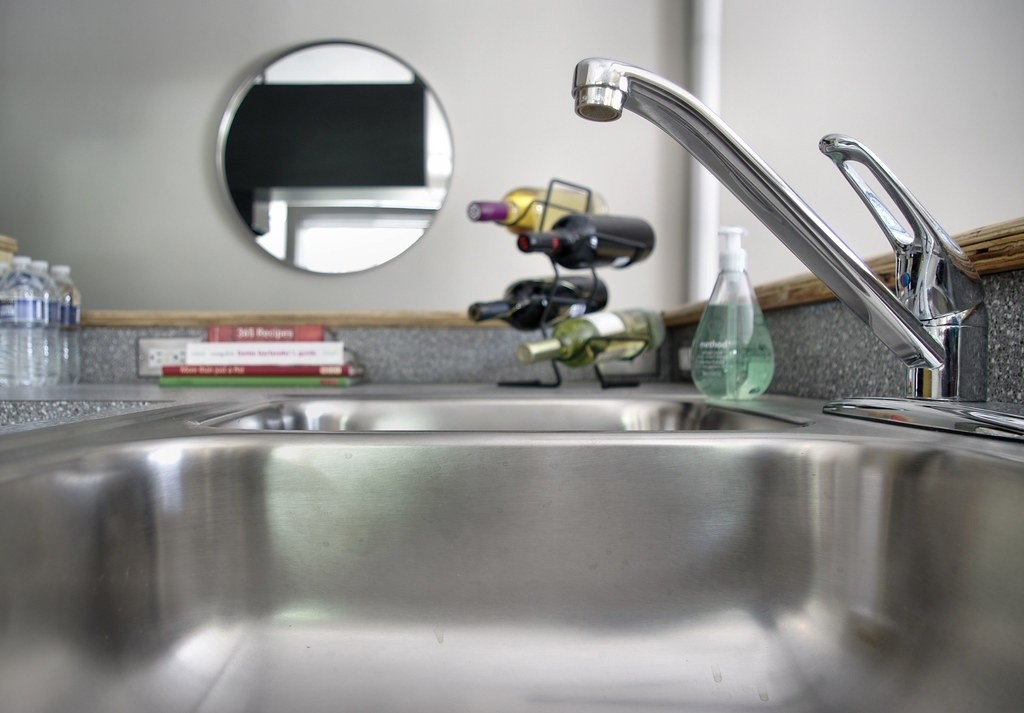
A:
[159,324,364,388]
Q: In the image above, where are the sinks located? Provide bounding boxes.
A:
[9,394,935,434]
[0,436,1024,713]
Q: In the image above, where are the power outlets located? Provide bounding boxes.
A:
[134,336,204,381]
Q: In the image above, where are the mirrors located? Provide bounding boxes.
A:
[218,42,456,276]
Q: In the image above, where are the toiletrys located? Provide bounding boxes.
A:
[689,224,775,401]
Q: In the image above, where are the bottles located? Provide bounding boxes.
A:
[516,308,666,369]
[0,255,82,386]
[467,188,607,234]
[518,213,655,270]
[469,275,608,331]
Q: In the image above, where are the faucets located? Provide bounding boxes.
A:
[572,57,990,401]
